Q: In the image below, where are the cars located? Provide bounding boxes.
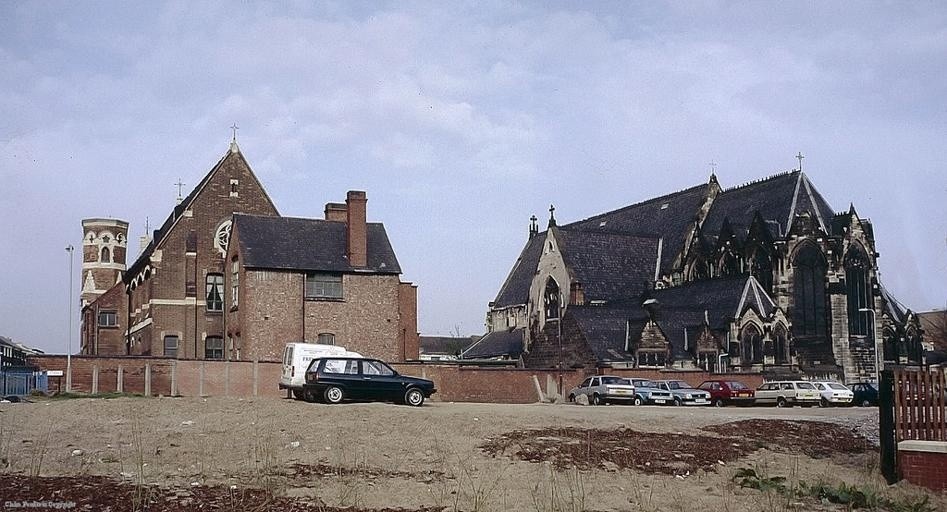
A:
[697,380,755,407]
[756,380,947,409]
[302,357,437,406]
[652,380,711,406]
[568,375,636,405]
[623,377,675,406]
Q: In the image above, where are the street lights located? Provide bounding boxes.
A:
[65,244,73,394]
[859,308,879,392]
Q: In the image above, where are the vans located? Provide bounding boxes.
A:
[279,342,380,400]
